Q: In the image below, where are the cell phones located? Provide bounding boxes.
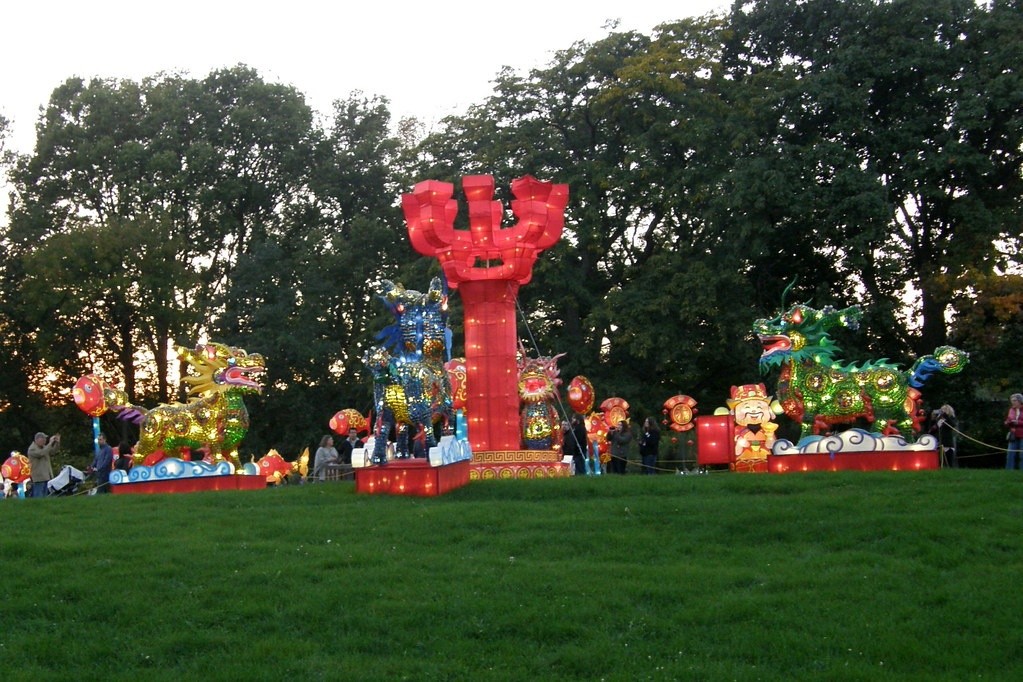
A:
[933,410,940,415]
[609,427,615,430]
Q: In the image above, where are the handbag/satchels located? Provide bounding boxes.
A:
[1006,431,1016,441]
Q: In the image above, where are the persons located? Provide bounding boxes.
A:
[336,428,364,481]
[314,435,339,482]
[561,412,587,475]
[25,482,33,497]
[1004,393,1023,470]
[606,420,632,475]
[637,416,660,475]
[27,432,61,497]
[715,383,786,461]
[114,441,133,473]
[87,433,112,494]
[931,404,957,468]
[6,483,18,498]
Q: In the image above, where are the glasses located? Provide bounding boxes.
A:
[1011,399,1019,402]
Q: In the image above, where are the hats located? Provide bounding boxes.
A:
[35,432,48,439]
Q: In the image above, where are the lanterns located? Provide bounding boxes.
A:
[693,409,698,423]
[672,437,694,446]
[662,409,668,425]
[917,399,925,416]
